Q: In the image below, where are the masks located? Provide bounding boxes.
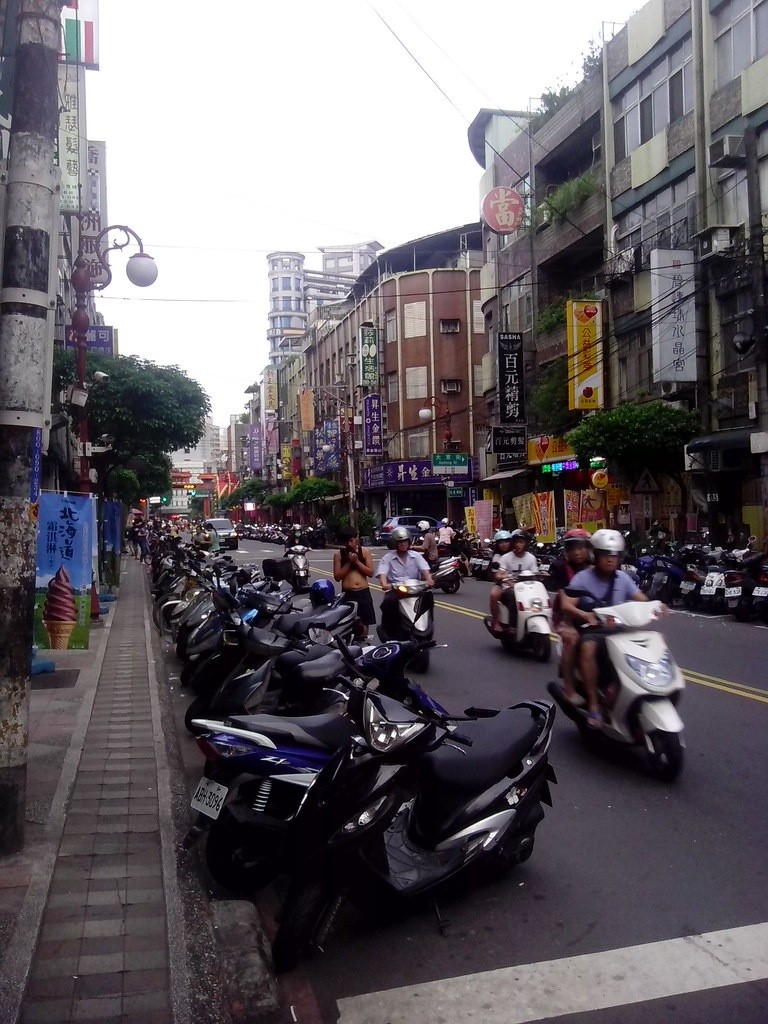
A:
[295,531,301,537]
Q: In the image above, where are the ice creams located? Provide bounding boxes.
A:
[41,563,78,650]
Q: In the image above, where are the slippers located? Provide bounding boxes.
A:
[585,709,604,730]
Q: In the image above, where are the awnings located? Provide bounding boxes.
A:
[687,427,762,467]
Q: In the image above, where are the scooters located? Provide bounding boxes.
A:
[546,587,689,777]
[145,524,768,971]
[374,574,451,672]
[482,561,556,662]
[281,546,315,589]
[408,544,466,594]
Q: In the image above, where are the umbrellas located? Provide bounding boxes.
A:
[131,508,144,516]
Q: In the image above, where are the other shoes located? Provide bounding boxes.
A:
[491,618,504,632]
[560,687,586,708]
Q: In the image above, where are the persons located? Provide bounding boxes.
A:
[200,523,220,551]
[128,510,244,566]
[549,528,592,707]
[284,523,312,552]
[376,526,434,641]
[333,524,377,635]
[316,517,322,528]
[437,517,472,576]
[410,520,438,563]
[489,530,512,633]
[494,529,545,631]
[563,529,670,728]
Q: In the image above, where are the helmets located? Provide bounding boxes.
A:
[391,527,413,542]
[562,528,591,541]
[493,530,511,540]
[294,524,302,530]
[309,579,336,606]
[416,520,432,532]
[511,528,531,539]
[589,529,626,556]
[449,520,456,525]
[441,517,449,525]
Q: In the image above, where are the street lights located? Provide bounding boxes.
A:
[418,397,458,528]
[70,222,157,633]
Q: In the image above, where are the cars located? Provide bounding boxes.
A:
[376,514,445,550]
[203,517,242,549]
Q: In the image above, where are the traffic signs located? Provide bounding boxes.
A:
[432,453,469,475]
